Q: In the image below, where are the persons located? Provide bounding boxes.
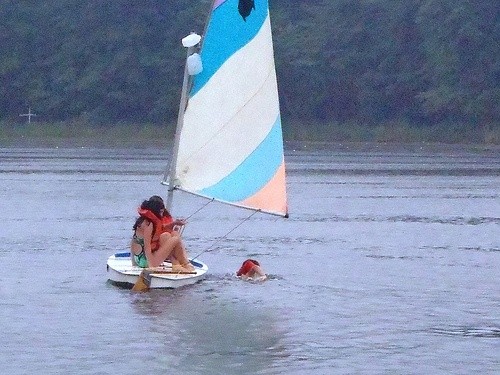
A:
[131,199,195,273]
[237,258,265,280]
[149,195,173,233]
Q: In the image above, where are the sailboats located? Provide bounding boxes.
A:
[108,0,290,292]
